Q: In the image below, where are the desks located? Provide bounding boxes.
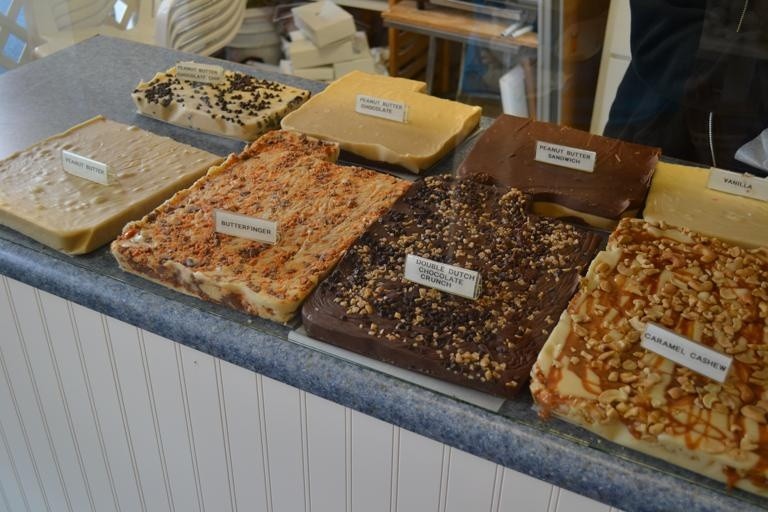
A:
[381,0,537,122]
[0,33,768,512]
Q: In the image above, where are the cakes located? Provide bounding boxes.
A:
[0,113,227,260]
[456,112,662,232]
[528,216,768,499]
[279,70,484,176]
[109,128,414,326]
[642,161,768,250]
[127,58,312,143]
[301,173,610,404]
